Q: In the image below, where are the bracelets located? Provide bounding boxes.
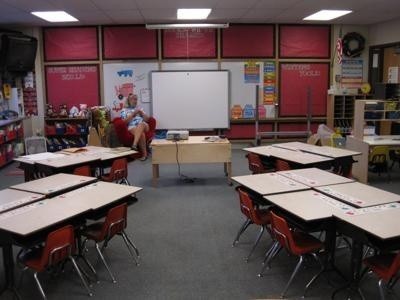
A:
[141,112,145,115]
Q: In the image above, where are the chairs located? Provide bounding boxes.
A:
[112,116,159,161]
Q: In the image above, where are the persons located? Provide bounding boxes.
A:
[120,94,150,161]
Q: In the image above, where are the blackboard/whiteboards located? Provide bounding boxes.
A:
[159,59,220,70]
[100,60,160,121]
[149,69,231,130]
[220,60,264,120]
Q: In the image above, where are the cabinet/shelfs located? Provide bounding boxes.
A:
[375,83,400,100]
[327,93,376,137]
[44,106,94,152]
[354,99,399,134]
[0,116,28,170]
[23,87,37,117]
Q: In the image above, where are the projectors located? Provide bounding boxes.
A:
[166,130,189,140]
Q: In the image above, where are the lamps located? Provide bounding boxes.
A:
[145,23,230,29]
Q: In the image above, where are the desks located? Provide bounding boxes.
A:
[230,136,400,300]
[0,145,143,300]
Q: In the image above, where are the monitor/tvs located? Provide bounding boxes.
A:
[0,29,37,80]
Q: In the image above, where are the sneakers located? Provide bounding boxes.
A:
[140,156,148,161]
[131,146,138,150]
